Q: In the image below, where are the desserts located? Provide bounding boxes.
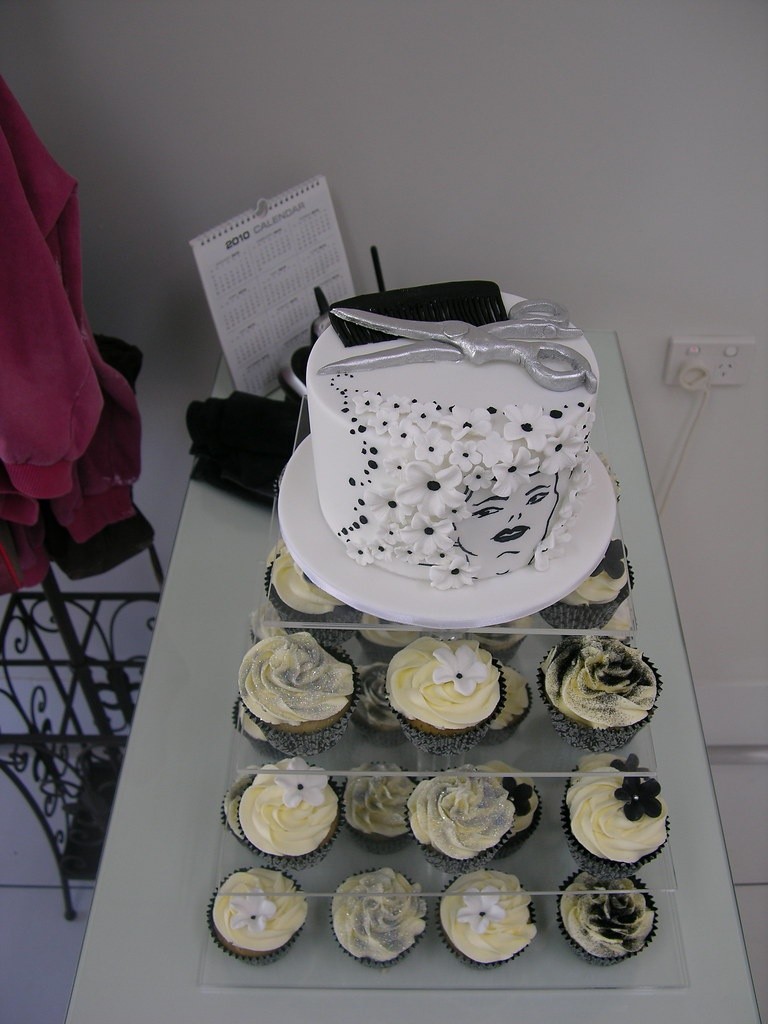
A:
[206,454,672,968]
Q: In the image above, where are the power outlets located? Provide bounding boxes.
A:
[663,336,755,386]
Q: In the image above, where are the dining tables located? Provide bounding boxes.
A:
[62,330,759,1023]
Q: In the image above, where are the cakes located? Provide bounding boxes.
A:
[306,280,600,591]
